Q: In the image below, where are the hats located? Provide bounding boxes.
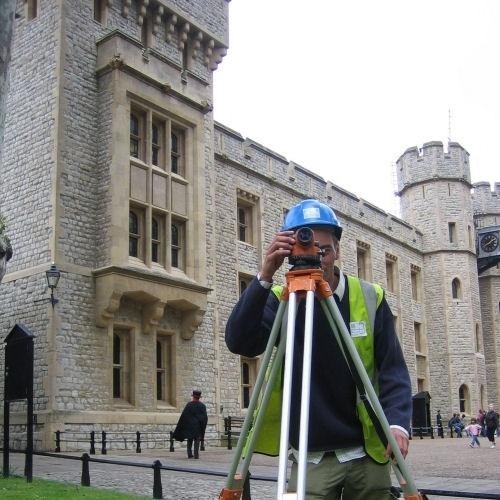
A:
[191,390,203,399]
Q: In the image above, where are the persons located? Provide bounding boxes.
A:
[172,390,208,459]
[224,199,413,500]
[436,403,500,448]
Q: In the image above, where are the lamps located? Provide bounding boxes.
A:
[45,264,61,311]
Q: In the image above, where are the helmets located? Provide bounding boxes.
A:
[281,198,342,231]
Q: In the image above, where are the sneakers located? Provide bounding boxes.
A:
[469,442,499,450]
[187,455,199,459]
